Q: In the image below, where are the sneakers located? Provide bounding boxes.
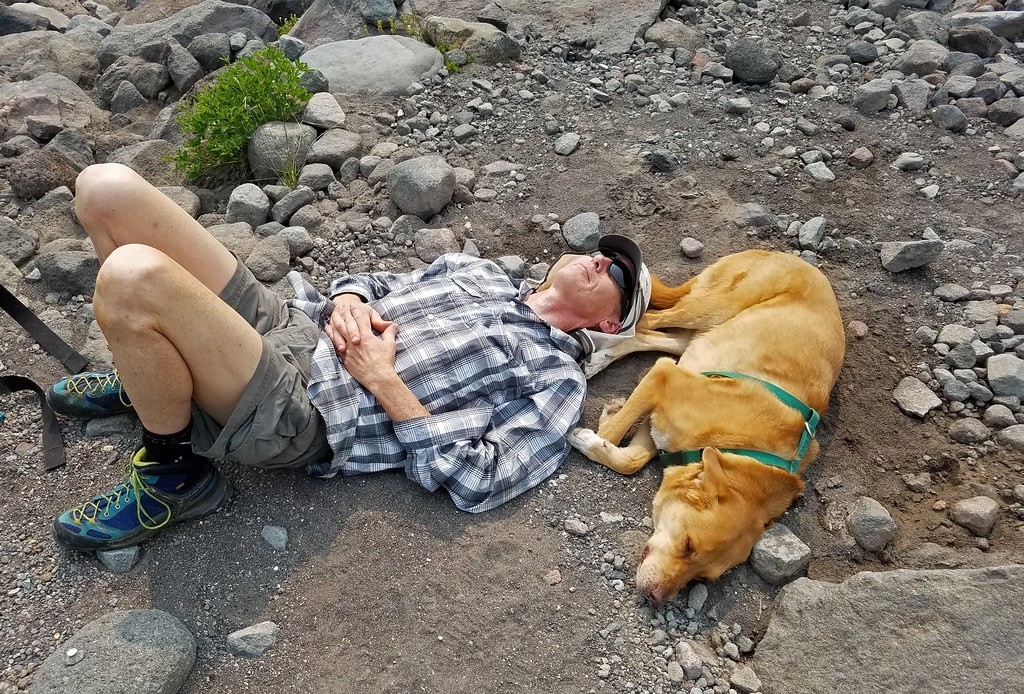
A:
[51,445,231,551]
[45,368,137,418]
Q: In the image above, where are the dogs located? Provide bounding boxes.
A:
[566,249,846,605]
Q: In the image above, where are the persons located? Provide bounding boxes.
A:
[46,163,652,552]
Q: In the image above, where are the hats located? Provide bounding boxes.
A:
[598,234,652,334]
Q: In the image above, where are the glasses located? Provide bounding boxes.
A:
[598,246,632,322]
